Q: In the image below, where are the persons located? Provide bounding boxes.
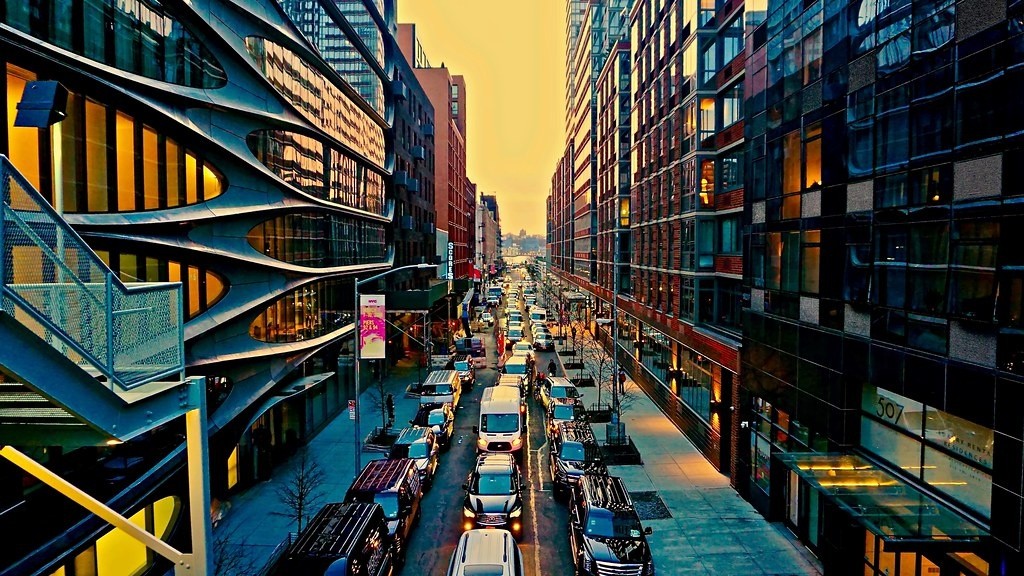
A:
[546,359,557,377]
[386,394,395,427]
[618,367,626,394]
[533,372,546,396]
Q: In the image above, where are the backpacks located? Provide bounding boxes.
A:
[619,371,626,382]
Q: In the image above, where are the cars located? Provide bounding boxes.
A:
[409,402,454,453]
[446,529,524,576]
[545,398,590,443]
[447,265,556,394]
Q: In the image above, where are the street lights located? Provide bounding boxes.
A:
[355,263,439,479]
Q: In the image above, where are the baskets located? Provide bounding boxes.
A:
[549,372,553,377]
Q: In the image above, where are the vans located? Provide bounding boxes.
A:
[473,386,527,462]
[550,422,608,501]
[272,502,396,576]
[344,458,420,551]
[570,475,654,576]
[384,427,440,493]
[539,376,584,413]
[462,453,526,542]
[419,370,462,418]
